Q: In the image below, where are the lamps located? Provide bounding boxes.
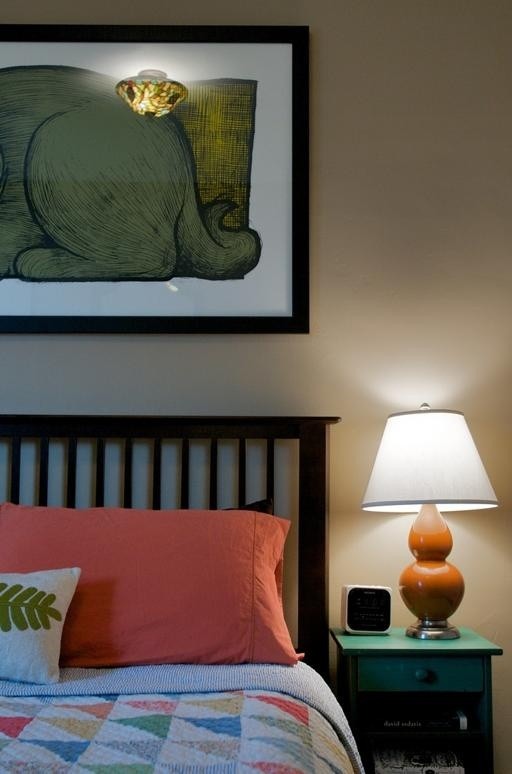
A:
[362,401,500,641]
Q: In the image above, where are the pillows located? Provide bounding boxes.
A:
[0,501,301,674]
[0,566,85,688]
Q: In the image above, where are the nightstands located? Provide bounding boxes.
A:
[326,626,504,774]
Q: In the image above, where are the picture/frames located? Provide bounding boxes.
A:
[0,23,311,335]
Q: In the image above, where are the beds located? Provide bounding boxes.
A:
[1,411,341,774]
